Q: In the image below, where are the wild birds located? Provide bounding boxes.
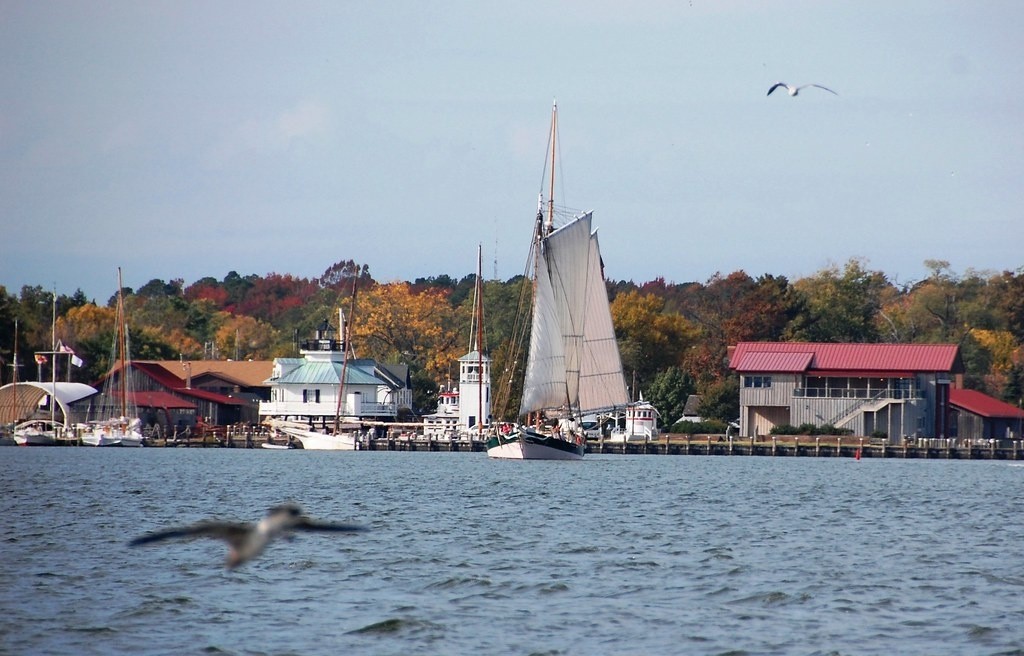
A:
[127,499,373,574]
[766,81,837,98]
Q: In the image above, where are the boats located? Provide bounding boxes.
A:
[14,419,80,446]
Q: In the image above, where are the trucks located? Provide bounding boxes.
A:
[577,414,600,442]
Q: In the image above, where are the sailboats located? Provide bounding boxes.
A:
[80,265,144,447]
[483,96,631,459]
[270,263,359,450]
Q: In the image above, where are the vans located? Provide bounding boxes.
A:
[466,421,519,438]
